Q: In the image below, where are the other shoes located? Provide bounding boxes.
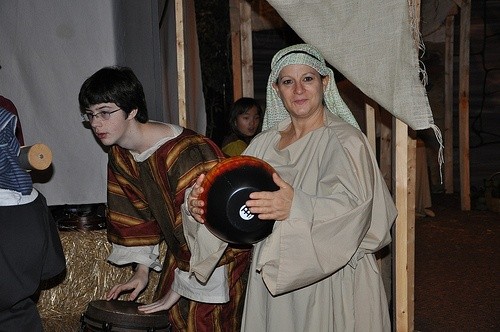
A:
[416,209,435,217]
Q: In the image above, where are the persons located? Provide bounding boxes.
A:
[0,96,68,332]
[181,44,399,332]
[221,97,263,157]
[78,65,253,332]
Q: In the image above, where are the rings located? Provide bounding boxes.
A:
[192,207,196,212]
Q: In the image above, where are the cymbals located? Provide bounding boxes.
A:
[57,217,106,231]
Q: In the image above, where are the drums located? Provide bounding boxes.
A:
[197,156,283,245]
[80,300,171,332]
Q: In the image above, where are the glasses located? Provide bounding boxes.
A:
[83,108,121,121]
